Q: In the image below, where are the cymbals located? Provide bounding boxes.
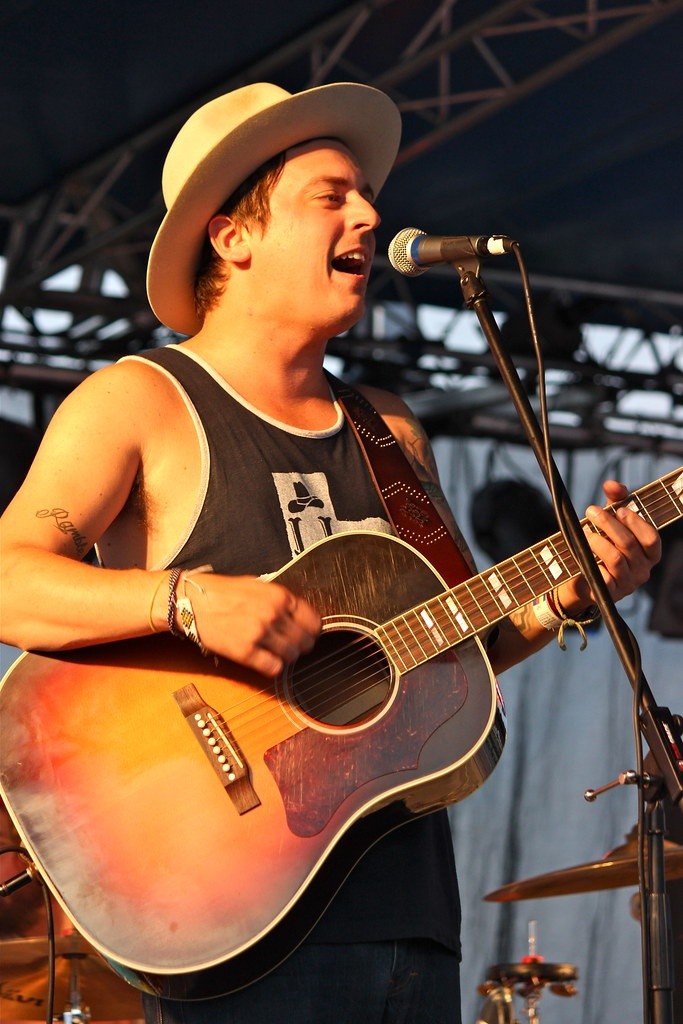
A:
[0,935,144,1024]
[482,833,683,903]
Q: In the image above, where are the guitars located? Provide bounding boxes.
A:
[0,467,683,1003]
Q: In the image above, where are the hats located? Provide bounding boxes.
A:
[146,82,402,339]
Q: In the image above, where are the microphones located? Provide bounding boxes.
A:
[387,226,513,278]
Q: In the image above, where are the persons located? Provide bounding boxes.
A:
[0,81,662,1024]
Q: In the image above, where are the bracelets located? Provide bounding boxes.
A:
[148,562,213,655]
[532,587,601,651]
